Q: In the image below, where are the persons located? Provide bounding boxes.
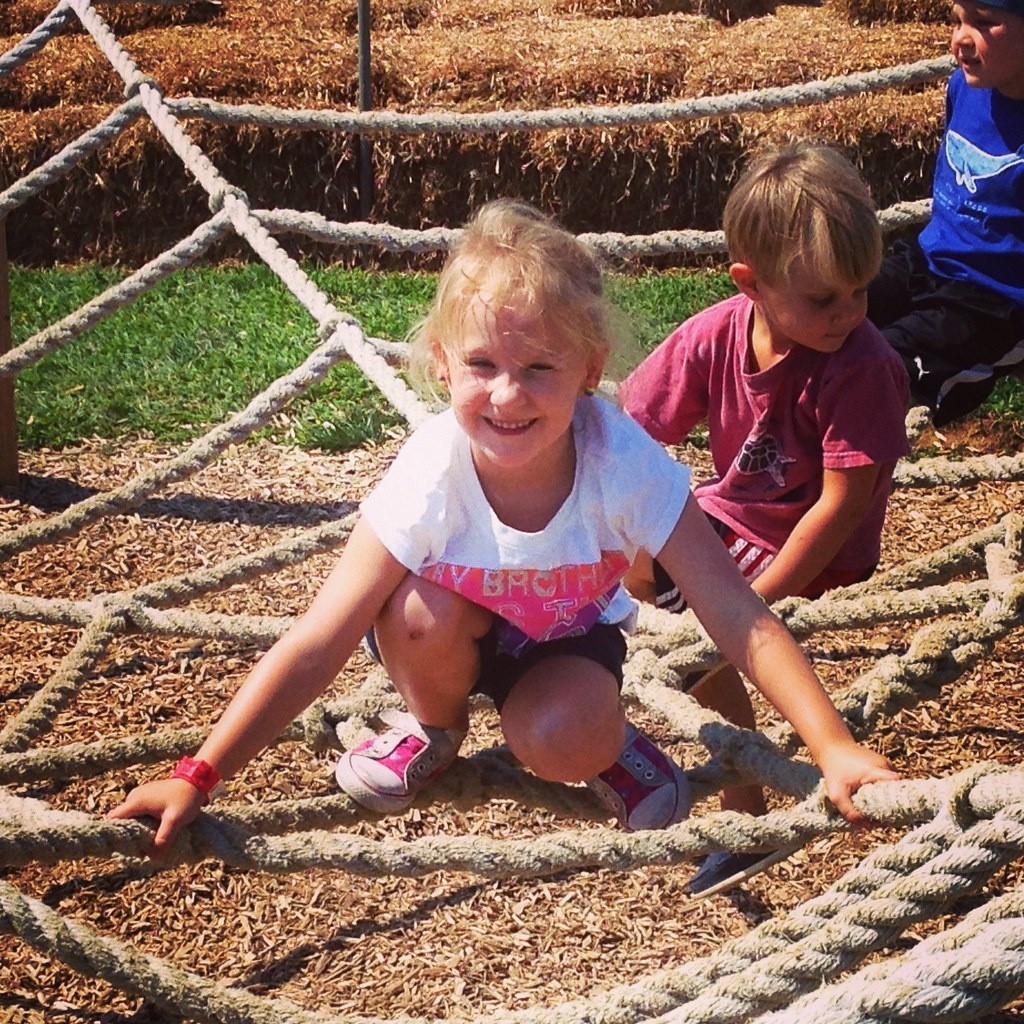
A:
[106,198,906,850]
[616,146,912,615]
[865,0,1024,426]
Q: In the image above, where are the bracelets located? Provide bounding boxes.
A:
[172,756,225,808]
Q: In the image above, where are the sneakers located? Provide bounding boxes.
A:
[334,707,470,814]
[585,719,693,832]
